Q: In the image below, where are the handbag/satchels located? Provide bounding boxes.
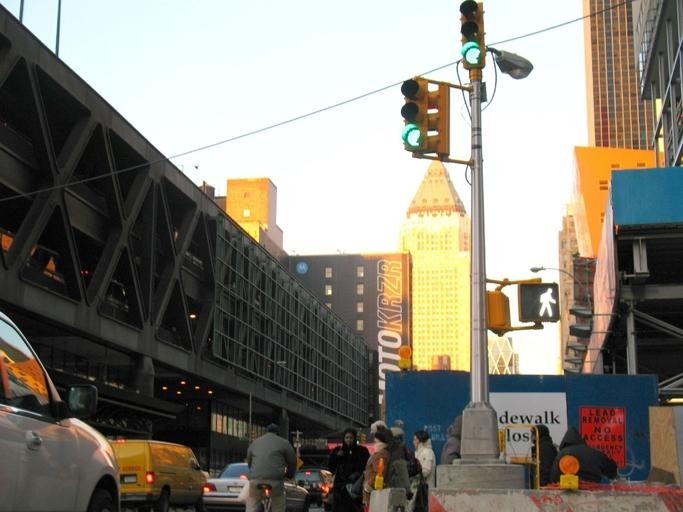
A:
[406,457,422,476]
[413,483,428,512]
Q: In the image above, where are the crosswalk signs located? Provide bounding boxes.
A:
[517,282,561,322]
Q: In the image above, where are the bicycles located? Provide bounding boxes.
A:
[256,483,272,512]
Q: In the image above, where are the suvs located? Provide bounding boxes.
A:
[294,467,334,507]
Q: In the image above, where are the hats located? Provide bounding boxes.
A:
[266,424,280,434]
[370,420,388,433]
[374,425,393,443]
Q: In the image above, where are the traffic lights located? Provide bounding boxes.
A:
[401,76,428,152]
[459,0,485,70]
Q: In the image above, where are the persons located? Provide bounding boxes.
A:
[440,414,462,465]
[243,423,297,512]
[548,426,619,481]
[530,424,557,489]
[328,419,436,512]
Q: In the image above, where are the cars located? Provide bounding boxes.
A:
[1,312,124,512]
[201,462,313,512]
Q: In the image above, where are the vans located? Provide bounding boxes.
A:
[108,437,209,512]
